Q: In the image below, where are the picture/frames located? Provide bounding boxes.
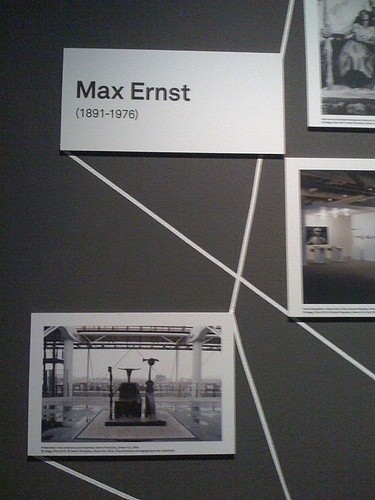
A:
[28,313,236,457]
[302,0,375,129]
[283,157,375,318]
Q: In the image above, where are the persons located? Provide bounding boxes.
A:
[338,11,375,86]
